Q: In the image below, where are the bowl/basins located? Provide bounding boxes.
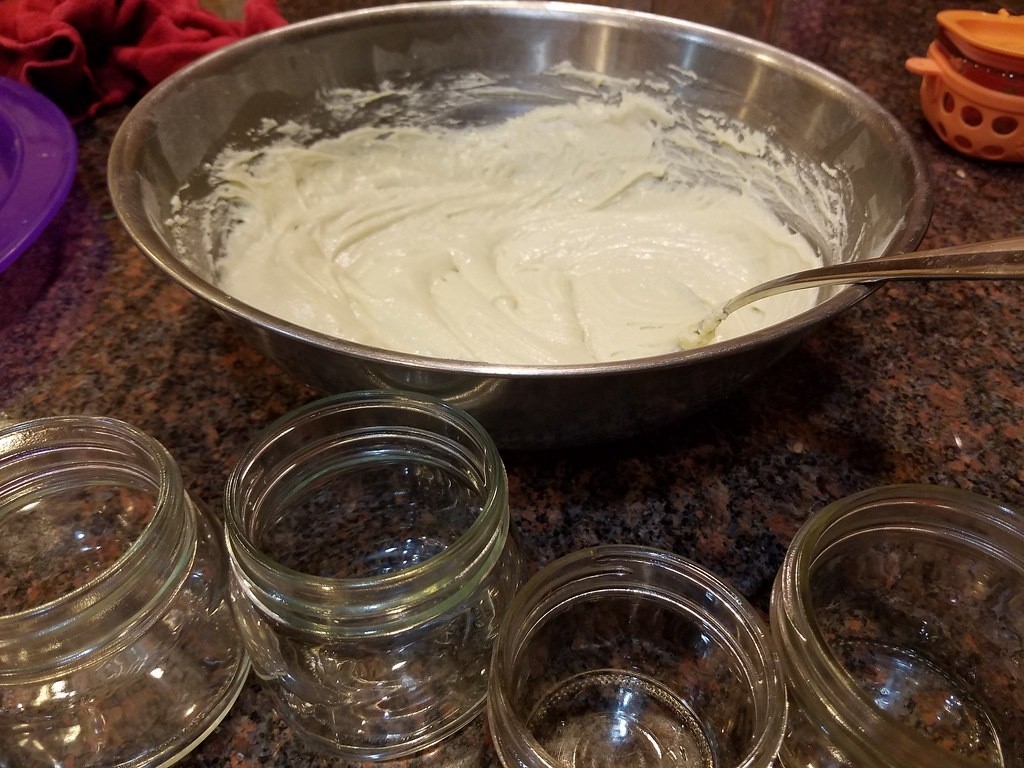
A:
[106,0,938,456]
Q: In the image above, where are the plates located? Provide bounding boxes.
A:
[0,76,77,275]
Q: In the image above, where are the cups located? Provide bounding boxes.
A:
[0,414,252,768]
[487,544,790,768]
[904,8,1024,164]
[226,389,527,764]
[769,484,1024,768]
